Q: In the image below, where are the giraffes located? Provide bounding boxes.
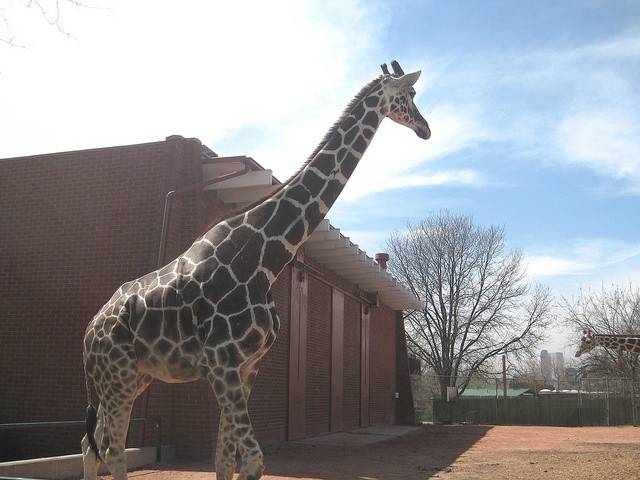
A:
[80,60,431,480]
[575,327,640,358]
